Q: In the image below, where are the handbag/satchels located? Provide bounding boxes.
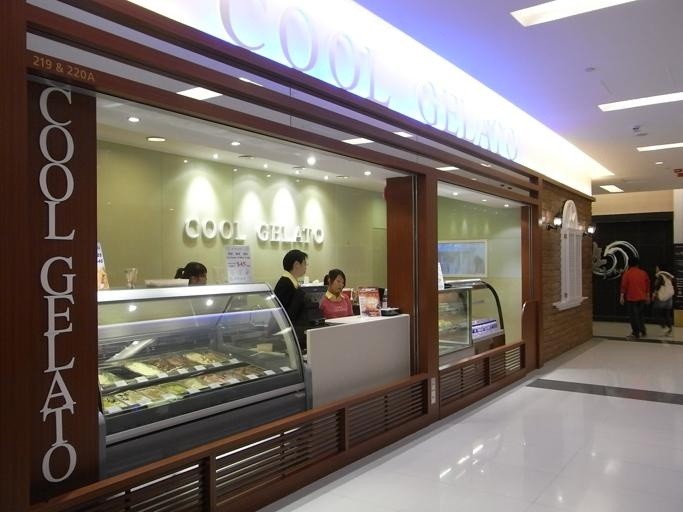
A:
[657,273,675,302]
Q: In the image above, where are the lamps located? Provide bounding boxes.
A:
[585,222,596,238]
[546,211,563,232]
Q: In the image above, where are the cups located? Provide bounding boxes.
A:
[303,276,309,284]
[125,268,137,289]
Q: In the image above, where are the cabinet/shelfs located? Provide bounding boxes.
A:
[99,283,309,446]
[438,280,506,368]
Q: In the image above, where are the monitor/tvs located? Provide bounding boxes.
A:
[298,286,327,314]
[439,239,488,279]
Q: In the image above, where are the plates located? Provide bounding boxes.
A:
[144,278,190,287]
[124,361,160,378]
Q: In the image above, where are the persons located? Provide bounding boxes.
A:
[618,257,653,342]
[266,249,321,353]
[172,261,244,339]
[318,268,353,318]
[650,261,678,337]
